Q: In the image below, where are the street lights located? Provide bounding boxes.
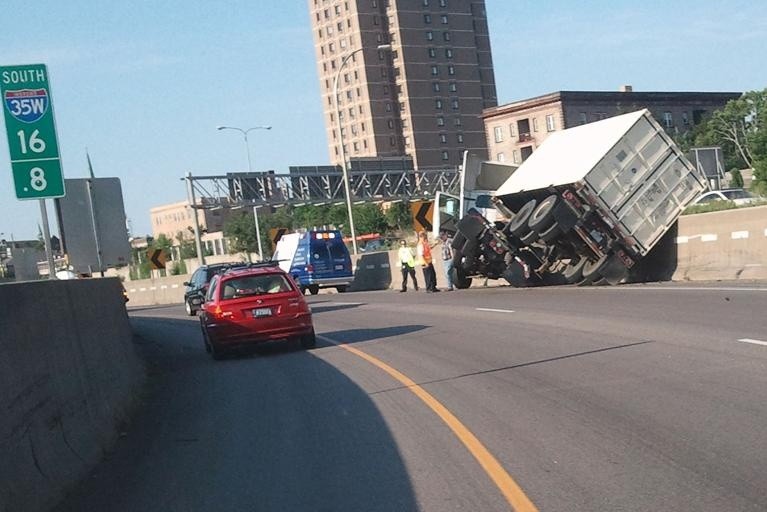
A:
[333,44,392,255]
[217,123,272,171]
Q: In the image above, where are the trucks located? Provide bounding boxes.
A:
[433,107,707,289]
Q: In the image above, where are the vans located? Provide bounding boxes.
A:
[269,229,355,295]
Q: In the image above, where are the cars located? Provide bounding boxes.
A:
[690,189,765,206]
[185,261,316,360]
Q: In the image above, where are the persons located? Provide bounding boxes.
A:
[395,240,418,293]
[438,233,456,291]
[415,230,441,293]
